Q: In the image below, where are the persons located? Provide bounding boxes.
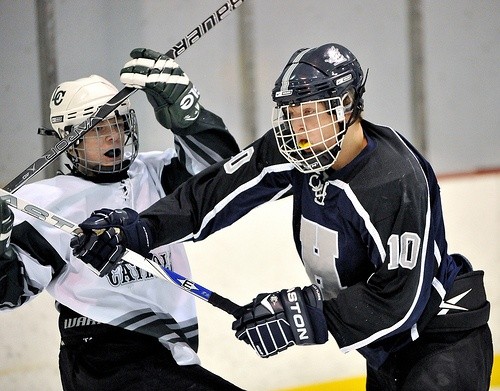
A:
[71,43,494,391]
[0,46,241,391]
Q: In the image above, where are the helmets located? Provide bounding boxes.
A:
[50,75,132,182]
[272,44,364,172]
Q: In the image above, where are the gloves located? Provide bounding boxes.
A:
[0,198,14,255]
[120,48,201,129]
[232,284,329,358]
[70,208,154,277]
